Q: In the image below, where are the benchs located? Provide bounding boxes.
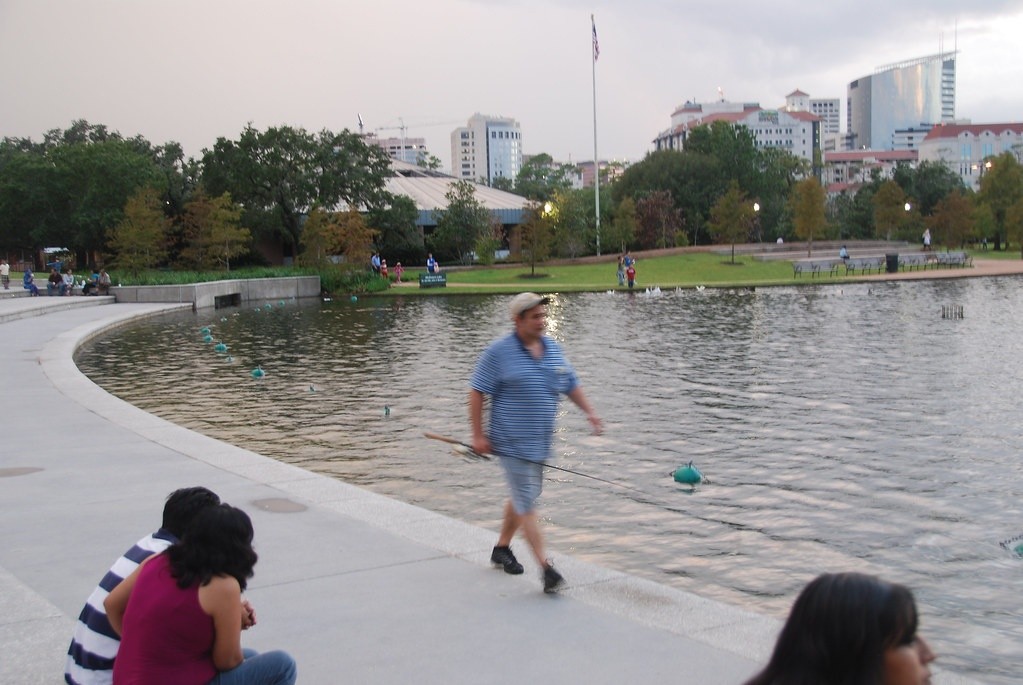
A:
[842,256,882,277]
[898,254,928,272]
[790,260,838,278]
[935,252,973,270]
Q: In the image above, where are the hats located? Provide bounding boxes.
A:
[509,291,551,317]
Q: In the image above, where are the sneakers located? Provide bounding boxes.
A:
[542,568,570,593]
[490,546,525,574]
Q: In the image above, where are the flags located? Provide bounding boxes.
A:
[592,20,600,60]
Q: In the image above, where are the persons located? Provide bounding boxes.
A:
[427,253,438,274]
[81,268,111,296]
[921,227,932,252]
[371,251,388,281]
[63,486,257,685]
[467,291,607,593]
[0,259,10,289]
[616,250,638,289]
[394,262,404,284]
[982,237,987,251]
[840,245,850,265]
[23,268,40,297]
[46,258,63,273]
[63,268,74,296]
[47,268,64,296]
[742,571,937,685]
[103,504,298,685]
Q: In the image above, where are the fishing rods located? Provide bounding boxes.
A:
[423,431,664,501]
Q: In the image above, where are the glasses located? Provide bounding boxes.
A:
[526,313,550,320]
